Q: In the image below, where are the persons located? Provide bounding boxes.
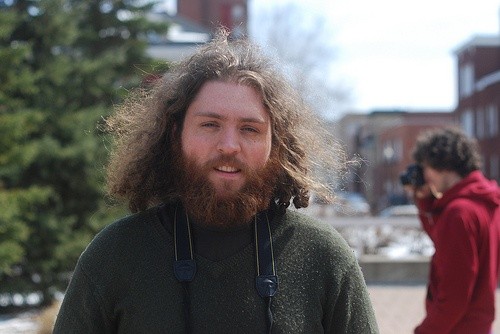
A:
[405,127,500,334]
[52,26,381,334]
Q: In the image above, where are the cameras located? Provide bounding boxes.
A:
[400,165,425,187]
